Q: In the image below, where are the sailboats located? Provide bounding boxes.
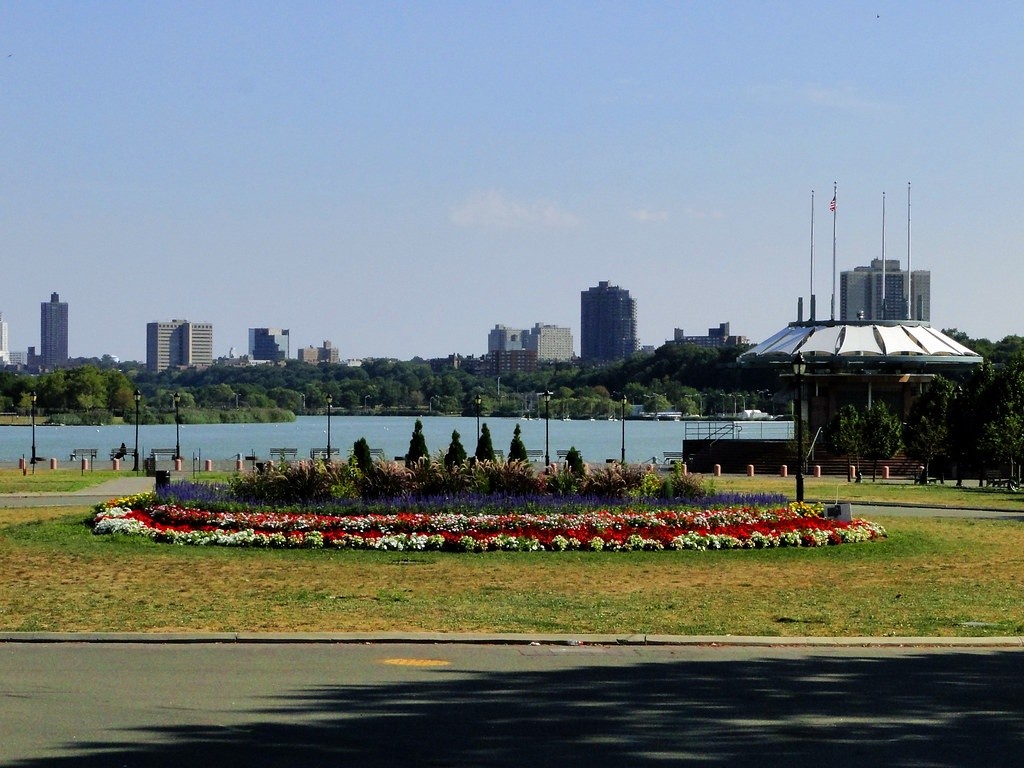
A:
[520,399,617,421]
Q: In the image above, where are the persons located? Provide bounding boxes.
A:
[112,443,127,461]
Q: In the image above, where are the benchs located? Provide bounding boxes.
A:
[269,448,298,458]
[73,449,97,458]
[986,470,1010,486]
[663,452,684,464]
[369,449,384,460]
[310,448,339,459]
[150,449,178,459]
[494,450,504,457]
[556,450,581,461]
[526,450,545,462]
[914,470,936,484]
[110,447,136,458]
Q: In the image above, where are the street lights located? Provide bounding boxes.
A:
[326,394,334,458]
[474,394,482,445]
[430,396,435,412]
[717,389,776,418]
[620,393,629,464]
[497,376,501,396]
[133,387,141,471]
[29,391,39,465]
[542,391,554,475]
[365,395,371,411]
[175,393,181,458]
[232,392,242,411]
[694,393,708,416]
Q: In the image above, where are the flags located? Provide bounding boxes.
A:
[830,197,836,211]
